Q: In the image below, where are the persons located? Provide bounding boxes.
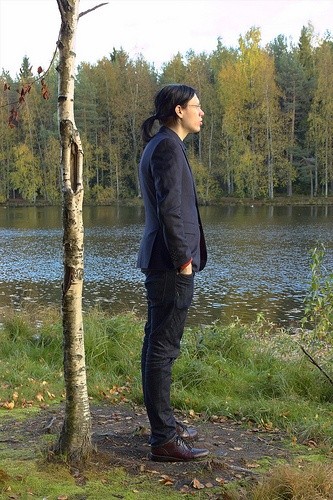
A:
[139,85,208,461]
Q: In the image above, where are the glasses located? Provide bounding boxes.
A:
[183,104,201,108]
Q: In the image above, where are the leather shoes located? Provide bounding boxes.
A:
[151,435,210,461]
[179,428,198,440]
